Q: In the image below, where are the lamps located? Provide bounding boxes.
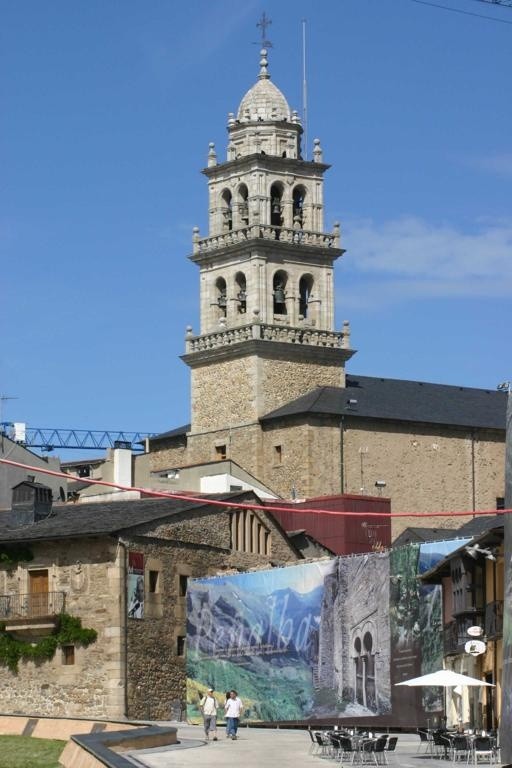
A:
[464,544,498,562]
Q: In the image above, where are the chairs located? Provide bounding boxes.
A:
[308,723,398,768]
[416,725,497,765]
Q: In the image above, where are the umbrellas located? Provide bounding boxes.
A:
[393,667,497,732]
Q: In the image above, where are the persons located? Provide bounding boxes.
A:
[225,691,231,736]
[127,574,144,617]
[224,690,243,739]
[199,688,219,740]
